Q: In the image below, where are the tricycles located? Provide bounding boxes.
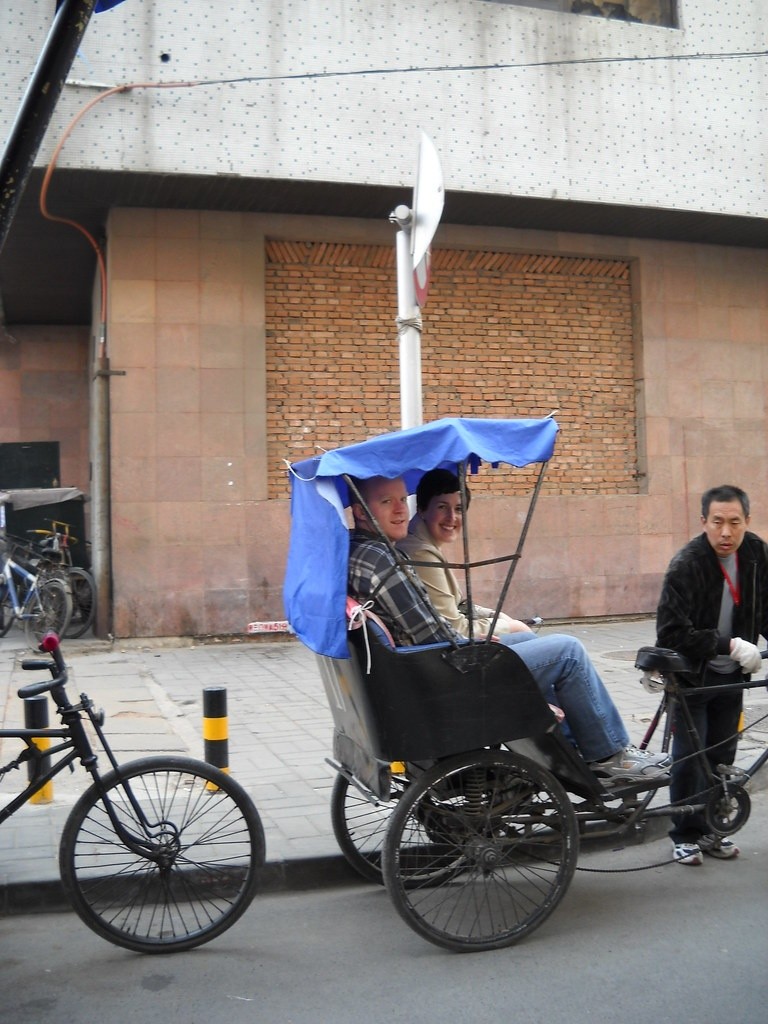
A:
[0,633,267,956]
[1,487,97,639]
[282,408,768,956]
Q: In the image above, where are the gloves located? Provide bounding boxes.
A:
[730,637,763,674]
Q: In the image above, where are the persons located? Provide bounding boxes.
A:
[346,471,673,787]
[395,467,531,638]
[656,485,767,865]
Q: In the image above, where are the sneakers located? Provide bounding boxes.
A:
[695,833,740,858]
[671,840,704,864]
[587,743,674,788]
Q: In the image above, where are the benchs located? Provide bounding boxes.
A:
[302,593,559,801]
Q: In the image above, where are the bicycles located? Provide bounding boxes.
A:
[0,532,74,654]
